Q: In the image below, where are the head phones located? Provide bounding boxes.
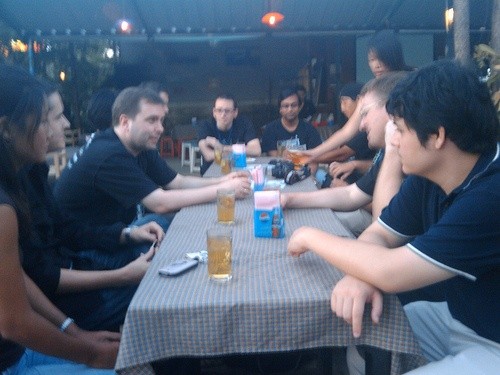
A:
[274,161,305,184]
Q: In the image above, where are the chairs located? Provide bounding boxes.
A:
[53,147,66,178]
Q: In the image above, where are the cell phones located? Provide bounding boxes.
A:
[158,259,198,276]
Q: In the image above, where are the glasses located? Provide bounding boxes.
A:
[212,108,232,116]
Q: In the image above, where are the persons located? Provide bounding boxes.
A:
[20,74,254,335]
[261,93,322,174]
[287,60,500,375]
[276,32,418,232]
[0,64,122,375]
[196,94,262,177]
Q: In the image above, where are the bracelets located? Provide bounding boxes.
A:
[61,318,75,333]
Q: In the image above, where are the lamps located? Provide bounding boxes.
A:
[261,3,283,25]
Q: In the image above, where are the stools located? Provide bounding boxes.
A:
[160,136,180,160]
[190,143,204,173]
[181,140,194,168]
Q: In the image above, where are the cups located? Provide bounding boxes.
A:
[277,138,306,171]
[214,143,233,174]
[217,188,235,225]
[206,226,233,281]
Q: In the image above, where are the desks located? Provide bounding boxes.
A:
[114,156,427,375]
